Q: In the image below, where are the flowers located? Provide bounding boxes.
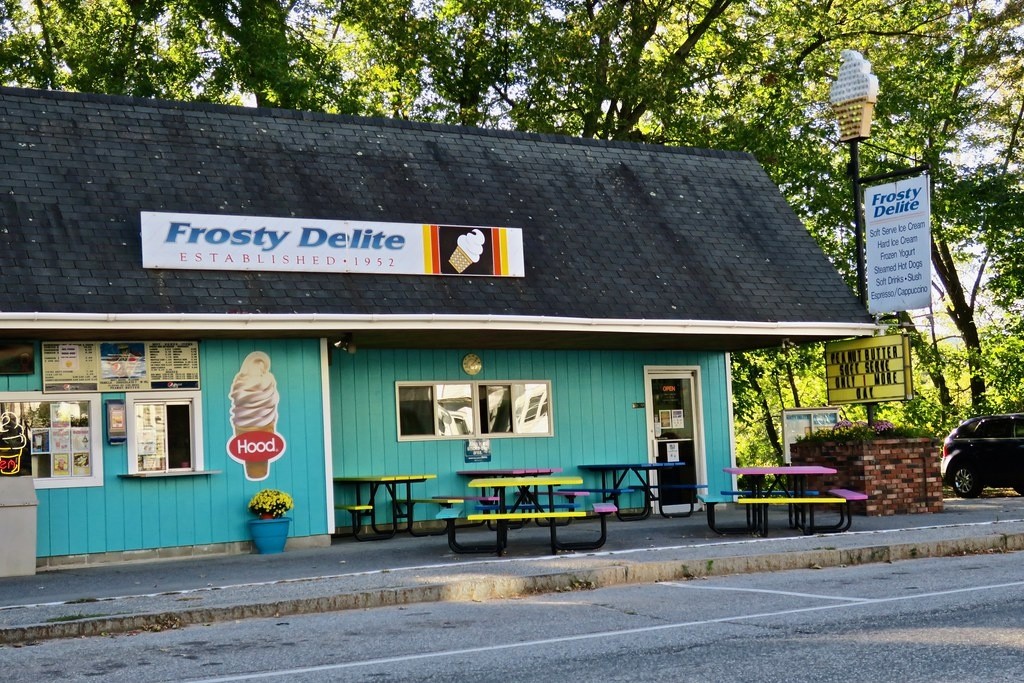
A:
[248,488,294,519]
[795,420,938,451]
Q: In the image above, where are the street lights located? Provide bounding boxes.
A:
[826,50,882,432]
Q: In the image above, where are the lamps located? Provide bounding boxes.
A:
[333,334,352,352]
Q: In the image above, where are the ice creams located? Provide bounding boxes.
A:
[229,351,278,480]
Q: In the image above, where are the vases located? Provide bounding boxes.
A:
[260,514,274,519]
[790,436,943,516]
[248,517,292,555]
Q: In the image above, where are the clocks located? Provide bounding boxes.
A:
[463,354,481,376]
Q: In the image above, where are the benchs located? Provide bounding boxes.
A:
[696,489,868,538]
[335,485,708,556]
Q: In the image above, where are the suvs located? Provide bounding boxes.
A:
[940,411,1024,498]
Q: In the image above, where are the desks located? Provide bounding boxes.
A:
[578,462,685,520]
[722,466,838,537]
[468,476,583,556]
[333,475,437,541]
[456,468,563,528]
